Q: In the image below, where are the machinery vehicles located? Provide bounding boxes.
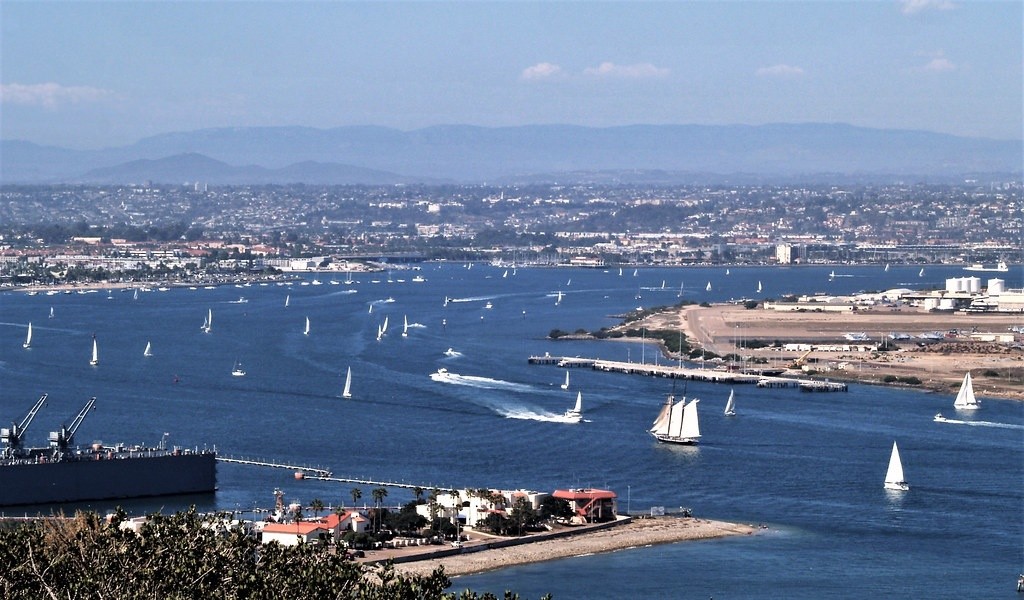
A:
[0,393,48,452]
[47,397,99,448]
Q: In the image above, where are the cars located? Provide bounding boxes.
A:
[383,536,445,548]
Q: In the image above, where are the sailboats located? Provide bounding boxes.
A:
[284,294,290,308]
[723,388,736,415]
[705,281,713,291]
[634,268,640,276]
[565,390,583,420]
[884,264,890,272]
[952,367,982,409]
[401,315,408,337]
[304,317,310,334]
[646,395,702,445]
[143,342,154,357]
[377,316,389,341]
[677,282,684,298]
[343,366,352,397]
[559,371,572,389]
[23,322,31,348]
[885,440,910,490]
[619,268,623,276]
[919,268,925,277]
[757,281,763,294]
[90,338,99,366]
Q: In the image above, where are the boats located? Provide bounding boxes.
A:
[28,288,99,296]
[429,368,461,383]
[119,279,430,302]
[934,412,947,421]
[232,369,246,376]
[962,260,1009,272]
